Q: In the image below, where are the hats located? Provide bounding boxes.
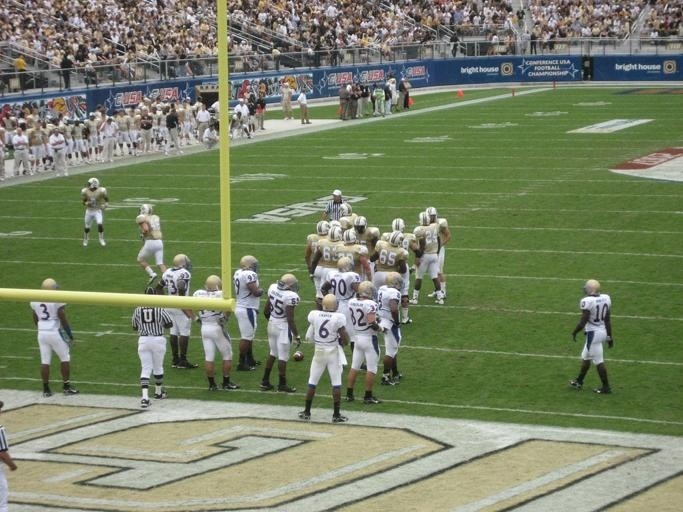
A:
[2,93,267,123]
[331,190,341,196]
[145,287,156,294]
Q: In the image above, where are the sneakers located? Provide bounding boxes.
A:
[140,399,152,408]
[43,393,54,399]
[568,380,583,390]
[208,360,297,393]
[1,127,265,182]
[177,362,198,368]
[345,372,402,404]
[83,239,88,247]
[99,239,106,246]
[298,411,311,420]
[592,388,611,394]
[340,112,392,120]
[63,388,79,396]
[147,273,157,286]
[154,391,168,401]
[402,292,446,324]
[332,416,347,422]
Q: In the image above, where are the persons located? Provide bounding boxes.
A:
[0,0,682,182]
[567,279,615,394]
[0,401,19,512]
[131,188,452,426]
[28,278,79,396]
[78,178,110,247]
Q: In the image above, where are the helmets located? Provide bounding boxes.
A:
[41,278,57,290]
[277,274,297,291]
[317,203,437,312]
[584,279,601,296]
[88,178,99,189]
[237,256,260,272]
[202,276,223,291]
[173,254,192,283]
[140,204,152,215]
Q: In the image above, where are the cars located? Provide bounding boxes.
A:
[208,99,253,121]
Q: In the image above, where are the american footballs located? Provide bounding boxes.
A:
[293,351,304,361]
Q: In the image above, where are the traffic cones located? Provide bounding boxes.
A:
[408,96,413,106]
[456,88,463,96]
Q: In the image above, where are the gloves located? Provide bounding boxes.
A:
[295,337,301,349]
